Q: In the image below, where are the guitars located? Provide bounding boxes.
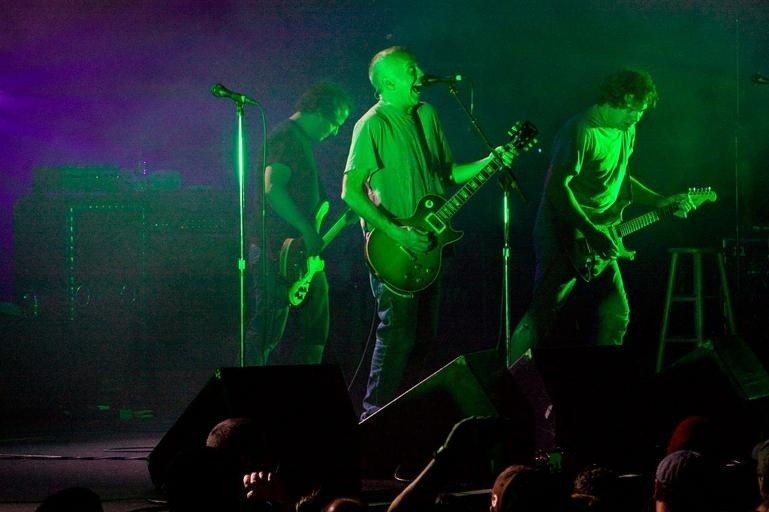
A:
[364,121,538,299]
[279,208,360,306]
[561,186,717,283]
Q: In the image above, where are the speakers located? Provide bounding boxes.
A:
[507,339,750,488]
[358,346,517,484]
[12,195,152,417]
[148,361,361,503]
[153,216,251,419]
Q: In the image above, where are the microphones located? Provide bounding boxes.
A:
[751,73,769,84]
[209,83,256,106]
[421,75,464,87]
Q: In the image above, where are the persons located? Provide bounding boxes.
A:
[334,40,520,424]
[258,80,355,366]
[534,62,702,351]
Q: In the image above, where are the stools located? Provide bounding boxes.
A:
[654,245,737,375]
